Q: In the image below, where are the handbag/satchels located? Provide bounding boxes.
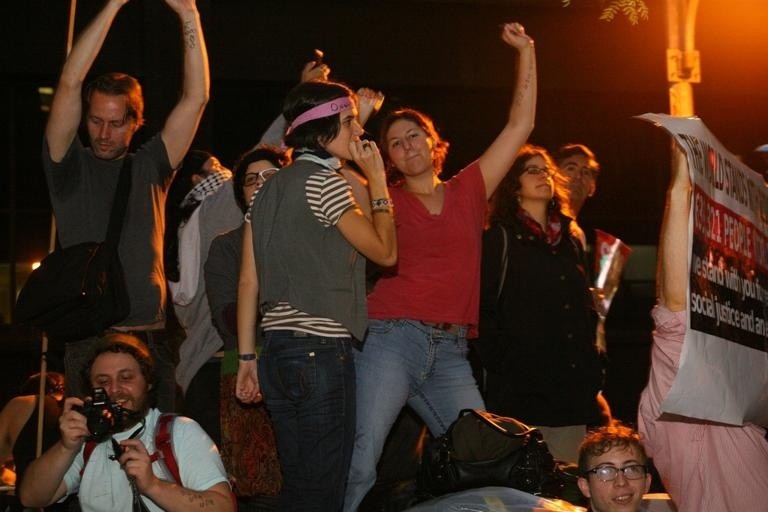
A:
[13,243,130,343]
[418,408,565,498]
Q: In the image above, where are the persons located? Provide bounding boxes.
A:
[17,331,240,511]
[38,0,214,326]
[168,18,768,511]
[0,371,66,512]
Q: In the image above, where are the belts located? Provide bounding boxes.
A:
[417,320,460,336]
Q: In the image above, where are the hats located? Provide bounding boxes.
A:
[87,335,153,369]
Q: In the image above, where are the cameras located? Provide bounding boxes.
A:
[72,387,126,443]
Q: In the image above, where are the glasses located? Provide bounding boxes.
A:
[579,466,648,481]
[239,168,280,186]
[520,167,557,176]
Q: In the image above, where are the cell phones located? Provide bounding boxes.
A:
[313,49,324,69]
